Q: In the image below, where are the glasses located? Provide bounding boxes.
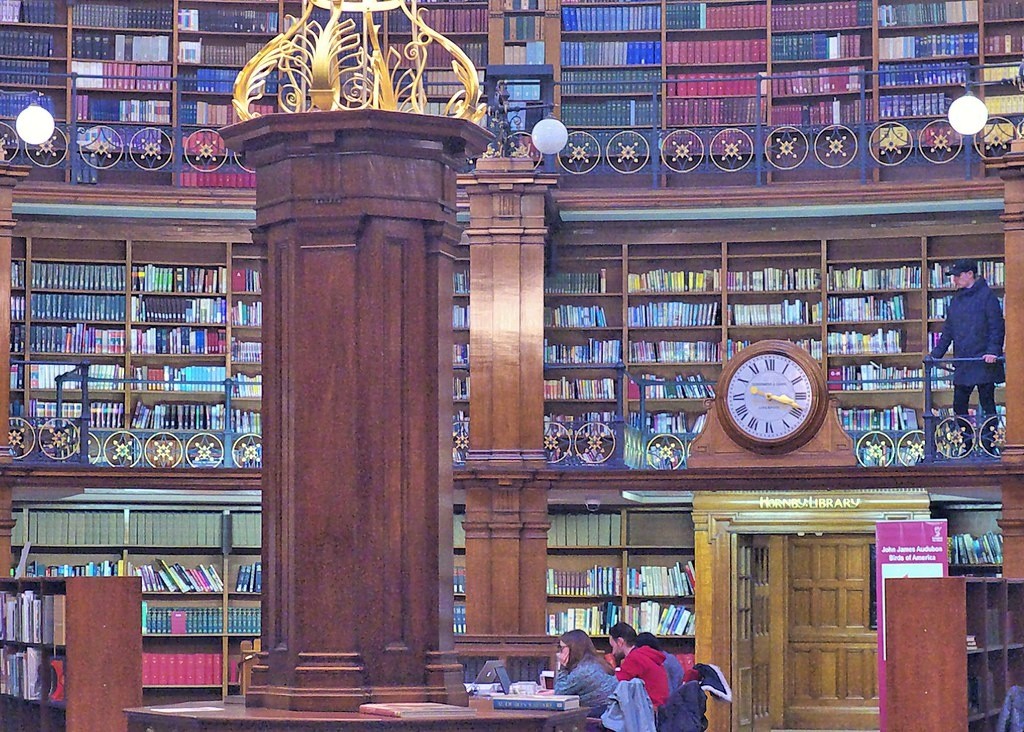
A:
[560,644,568,650]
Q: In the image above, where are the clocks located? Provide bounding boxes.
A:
[715,340,830,455]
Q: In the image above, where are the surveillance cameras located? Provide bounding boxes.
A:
[585,500,600,511]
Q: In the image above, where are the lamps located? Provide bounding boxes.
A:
[948,74,1024,136]
[484,101,568,154]
[0,88,55,144]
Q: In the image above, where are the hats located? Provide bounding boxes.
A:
[946,259,977,278]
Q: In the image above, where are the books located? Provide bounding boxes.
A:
[71,3,173,184]
[0,590,47,703]
[359,701,478,718]
[771,0,874,127]
[492,694,581,711]
[0,0,68,148]
[927,260,1006,391]
[561,0,664,156]
[5,261,262,469]
[878,0,980,147]
[282,0,490,128]
[726,266,824,360]
[944,532,1002,647]
[827,264,925,429]
[503,0,547,64]
[451,511,695,635]
[176,7,279,189]
[666,0,767,153]
[449,270,470,455]
[9,511,264,683]
[983,0,1024,143]
[544,270,721,435]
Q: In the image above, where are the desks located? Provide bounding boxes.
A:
[122,703,579,732]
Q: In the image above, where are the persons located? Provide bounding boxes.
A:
[926,258,1008,458]
[549,616,683,732]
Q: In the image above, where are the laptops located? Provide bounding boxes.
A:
[473,660,504,685]
[495,665,510,696]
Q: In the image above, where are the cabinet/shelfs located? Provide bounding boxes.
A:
[0,0,1024,732]
